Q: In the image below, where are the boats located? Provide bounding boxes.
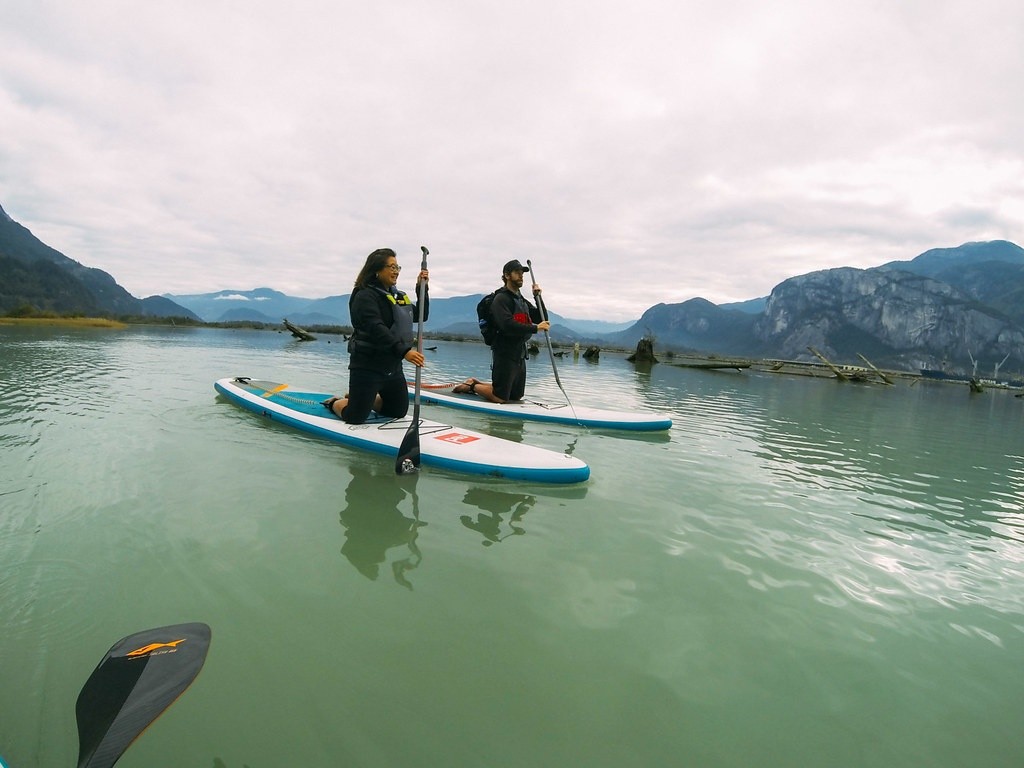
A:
[761,357,873,381]
[918,368,1013,390]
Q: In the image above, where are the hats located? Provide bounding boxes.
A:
[503,260,530,272]
[386,264,402,272]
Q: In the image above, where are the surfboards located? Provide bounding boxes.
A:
[403,385,674,432]
[216,377,590,482]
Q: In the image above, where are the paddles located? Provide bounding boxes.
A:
[524,258,571,406]
[397,245,430,478]
[67,619,219,768]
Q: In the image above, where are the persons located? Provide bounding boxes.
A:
[323,248,430,425]
[452,260,551,404]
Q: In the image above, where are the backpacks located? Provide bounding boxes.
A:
[477,293,496,346]
